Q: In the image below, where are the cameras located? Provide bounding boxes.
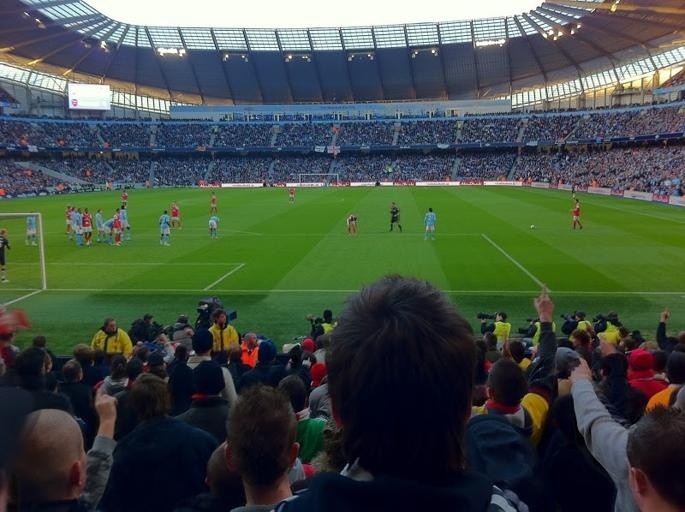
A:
[307,313,322,323]
[477,311,497,320]
[561,311,575,319]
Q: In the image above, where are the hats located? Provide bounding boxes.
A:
[302,338,315,352]
[278,374,307,400]
[189,360,226,395]
[552,346,581,379]
[258,342,277,363]
[626,348,656,380]
[309,362,328,387]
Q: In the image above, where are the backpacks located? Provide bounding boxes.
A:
[463,414,533,485]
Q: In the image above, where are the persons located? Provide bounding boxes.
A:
[1,229,9,271]
[1,273,685,511]
[2,100,684,245]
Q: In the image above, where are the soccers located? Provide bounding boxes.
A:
[531,224,536,229]
